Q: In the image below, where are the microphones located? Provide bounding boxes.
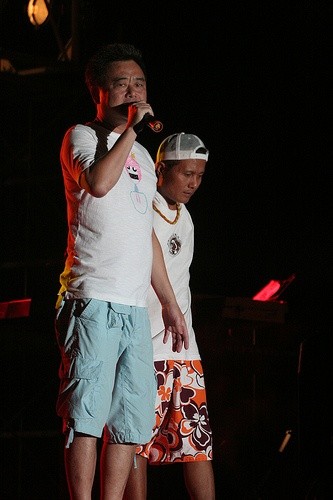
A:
[142,112,163,133]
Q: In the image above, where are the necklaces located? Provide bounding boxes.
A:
[151,201,182,225]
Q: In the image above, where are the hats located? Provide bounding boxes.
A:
[155,132,211,170]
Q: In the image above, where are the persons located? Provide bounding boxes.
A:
[123,133,233,500]
[59,41,192,500]
